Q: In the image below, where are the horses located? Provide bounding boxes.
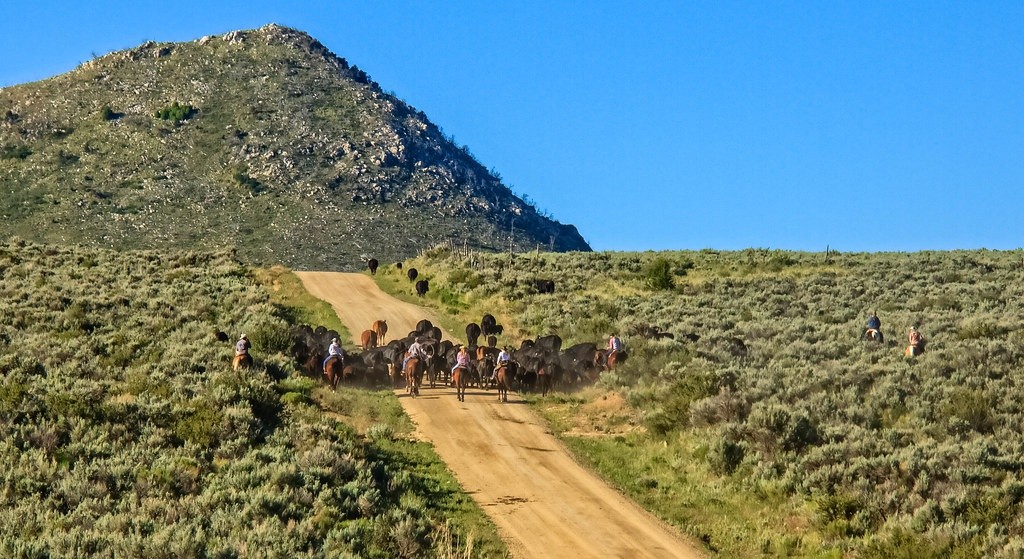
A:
[494,360,517,402]
[453,363,471,400]
[232,353,253,372]
[325,357,344,393]
[864,327,884,343]
[593,350,627,372]
[905,336,928,359]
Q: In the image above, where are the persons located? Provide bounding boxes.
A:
[322,337,345,374]
[490,346,511,380]
[603,333,621,366]
[451,346,473,382]
[401,337,433,375]
[235,334,254,366]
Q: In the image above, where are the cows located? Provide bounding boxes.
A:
[397,262,429,298]
[403,352,423,399]
[643,325,747,349]
[295,314,600,397]
[535,280,555,292]
[216,332,229,343]
[368,258,379,276]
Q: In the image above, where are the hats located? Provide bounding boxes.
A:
[501,346,508,351]
[240,334,246,339]
[415,337,419,341]
[459,346,467,349]
[331,338,337,342]
[610,333,614,336]
